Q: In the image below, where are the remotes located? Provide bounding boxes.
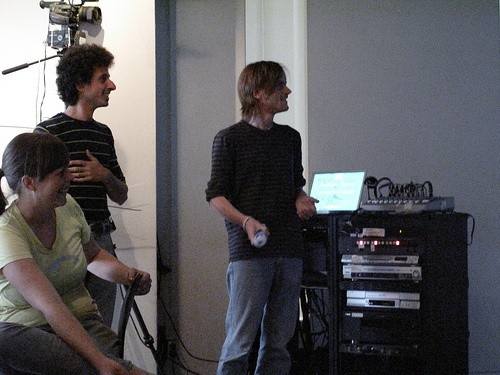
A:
[253,223,267,248]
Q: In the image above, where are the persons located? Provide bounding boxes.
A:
[204,61,319,375]
[33,44,127,329]
[0,132,156,375]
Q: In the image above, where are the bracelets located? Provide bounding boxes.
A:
[243,216,253,232]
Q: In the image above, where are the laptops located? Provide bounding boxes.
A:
[308,169,367,215]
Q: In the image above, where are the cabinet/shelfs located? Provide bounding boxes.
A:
[286,211,469,374]
